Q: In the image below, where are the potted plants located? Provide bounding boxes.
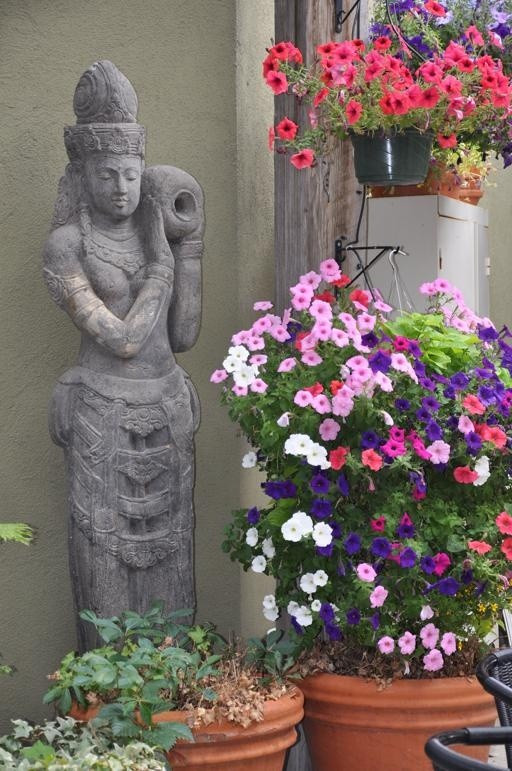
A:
[41,603,305,771]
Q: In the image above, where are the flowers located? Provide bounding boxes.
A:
[209,258,512,675]
[245,0,512,169]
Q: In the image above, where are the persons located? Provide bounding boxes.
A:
[43,59,205,654]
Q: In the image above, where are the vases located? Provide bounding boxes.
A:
[350,128,431,185]
[288,658,497,771]
[368,155,487,201]
